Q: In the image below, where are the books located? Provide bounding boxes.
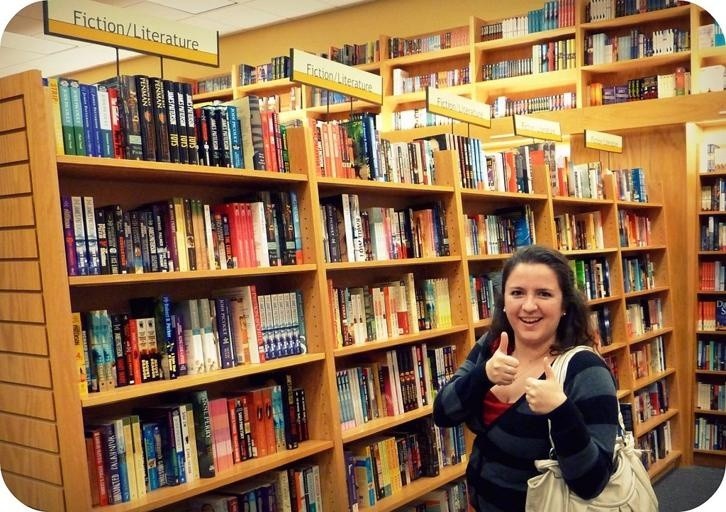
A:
[64,193,678,512]
[42,2,726,202]
[694,143,725,454]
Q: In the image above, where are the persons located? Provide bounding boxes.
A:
[432,246,618,509]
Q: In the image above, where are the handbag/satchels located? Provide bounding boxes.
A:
[525,432,661,512]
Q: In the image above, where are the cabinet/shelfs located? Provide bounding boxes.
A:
[1,0,726,512]
